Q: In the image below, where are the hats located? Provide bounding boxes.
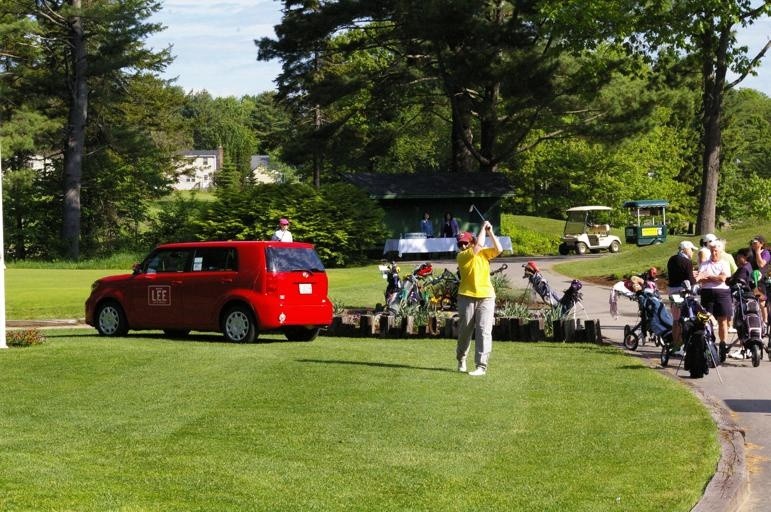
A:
[752,235,766,243]
[456,231,473,244]
[703,233,719,242]
[280,218,288,225]
[678,240,698,250]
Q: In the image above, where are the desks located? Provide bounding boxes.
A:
[381,234,515,261]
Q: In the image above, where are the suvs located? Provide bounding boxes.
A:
[84,240,334,342]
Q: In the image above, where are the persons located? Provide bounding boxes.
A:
[420,213,435,259]
[455,221,503,375]
[610,233,770,359]
[441,211,459,256]
[271,219,294,242]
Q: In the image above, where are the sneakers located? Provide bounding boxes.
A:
[469,366,486,376]
[457,359,468,372]
[718,340,753,361]
[674,349,687,357]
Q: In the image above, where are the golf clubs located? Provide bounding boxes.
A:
[732,283,744,317]
[469,205,490,230]
[522,264,534,277]
[679,280,700,318]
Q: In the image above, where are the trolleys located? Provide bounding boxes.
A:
[440,271,458,310]
[611,268,771,372]
